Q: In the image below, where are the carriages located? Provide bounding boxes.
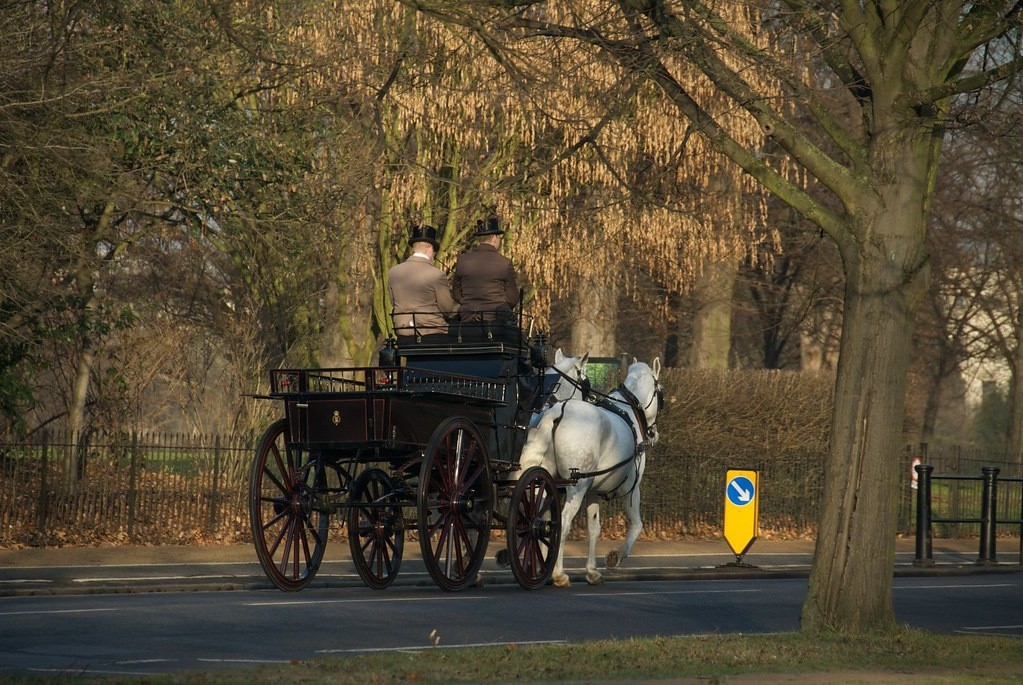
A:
[239,288,664,593]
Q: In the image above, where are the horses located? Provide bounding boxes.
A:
[449,348,595,587]
[505,355,663,590]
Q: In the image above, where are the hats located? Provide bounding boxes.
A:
[408,225,439,252]
[473,218,504,236]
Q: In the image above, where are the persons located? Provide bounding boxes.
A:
[387,224,459,337]
[451,218,520,324]
